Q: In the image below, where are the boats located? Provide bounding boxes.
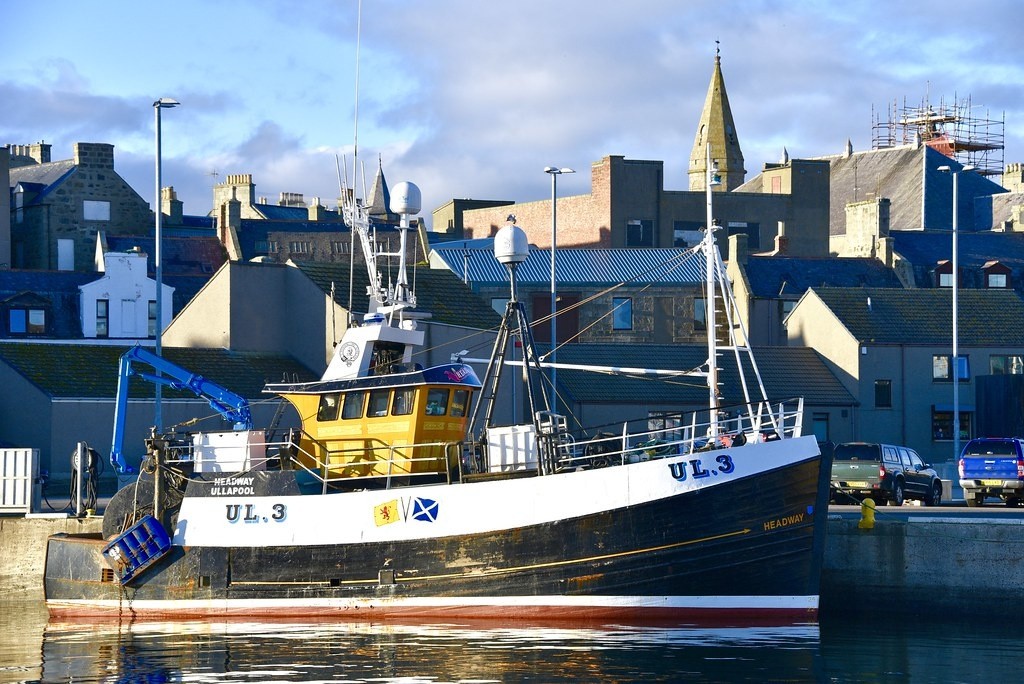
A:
[41,0,824,620]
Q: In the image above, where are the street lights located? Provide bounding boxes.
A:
[544,166,577,432]
[154,96,182,428]
[938,164,982,461]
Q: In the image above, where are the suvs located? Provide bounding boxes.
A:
[958,436,1024,507]
[829,441,943,506]
